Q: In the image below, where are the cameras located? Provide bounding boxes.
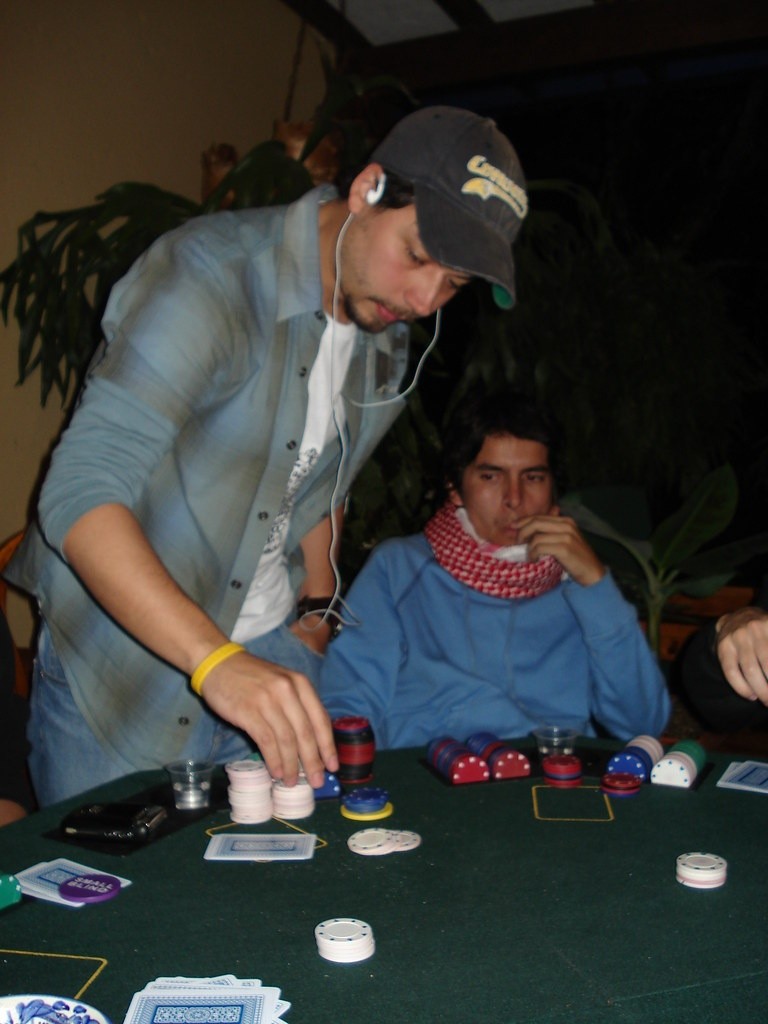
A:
[64,801,168,852]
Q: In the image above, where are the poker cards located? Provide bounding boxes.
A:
[118,971,298,1024]
[715,759,768,795]
[11,855,136,910]
[203,830,320,864]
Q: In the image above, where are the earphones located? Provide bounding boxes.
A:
[366,175,386,206]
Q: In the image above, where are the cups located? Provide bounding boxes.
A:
[166,759,215,809]
[533,723,579,772]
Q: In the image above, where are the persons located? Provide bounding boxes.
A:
[320,380,671,750]
[671,553,768,736]
[0,106,529,810]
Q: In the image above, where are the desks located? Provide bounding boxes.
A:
[0,735,768,1024]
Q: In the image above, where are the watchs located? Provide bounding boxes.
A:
[296,597,343,643]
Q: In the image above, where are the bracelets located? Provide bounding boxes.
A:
[189,642,246,697]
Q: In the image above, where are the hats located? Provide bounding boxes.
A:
[371,105,529,310]
